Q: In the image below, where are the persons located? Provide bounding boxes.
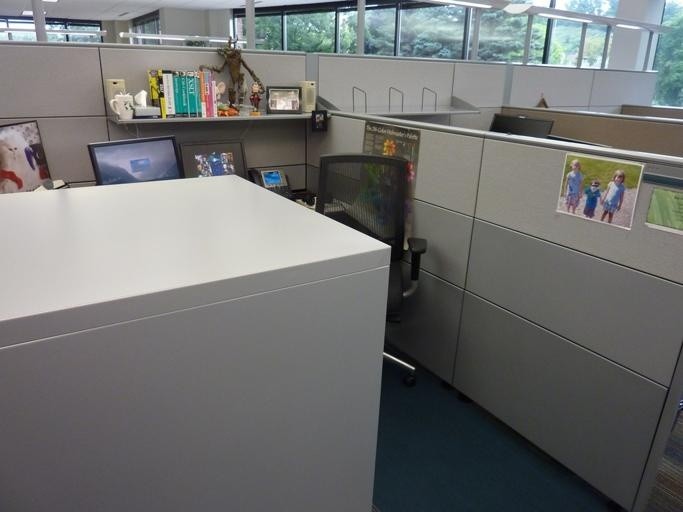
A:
[581,179,602,219]
[599,169,625,224]
[194,151,233,178]
[562,159,582,215]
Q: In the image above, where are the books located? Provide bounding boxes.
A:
[146,67,219,120]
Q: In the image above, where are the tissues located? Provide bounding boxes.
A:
[132,91,160,118]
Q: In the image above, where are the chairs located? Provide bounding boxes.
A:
[314,154,429,388]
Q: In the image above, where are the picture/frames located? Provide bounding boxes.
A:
[266,85,303,114]
[0,119,52,194]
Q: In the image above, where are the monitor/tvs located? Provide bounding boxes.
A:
[488,112,555,138]
[87,135,183,185]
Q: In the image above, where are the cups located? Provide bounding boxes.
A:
[109,95,133,119]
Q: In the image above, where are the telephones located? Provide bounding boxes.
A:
[248,168,290,194]
